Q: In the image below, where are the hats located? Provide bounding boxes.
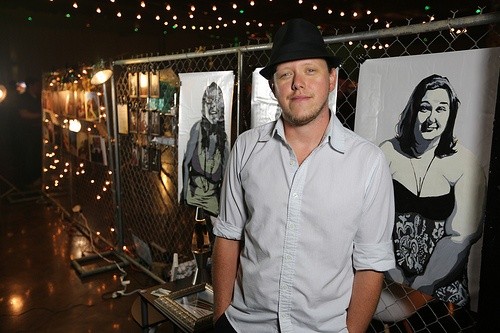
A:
[258,19,340,80]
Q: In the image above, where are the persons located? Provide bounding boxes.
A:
[181,81,231,216]
[210,43,396,333]
[376,74,487,308]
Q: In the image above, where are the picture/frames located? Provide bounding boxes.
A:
[154,282,217,331]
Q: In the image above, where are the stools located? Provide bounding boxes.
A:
[138,277,205,333]
